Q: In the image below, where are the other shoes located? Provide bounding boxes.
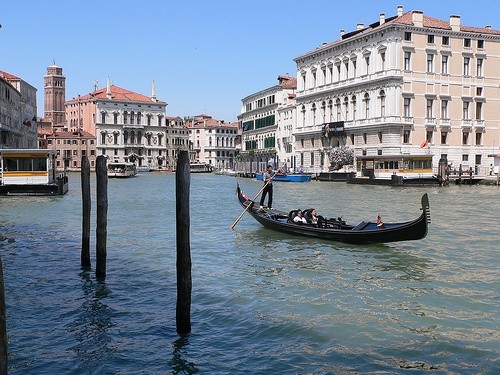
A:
[260,206,263,209]
[267,208,273,210]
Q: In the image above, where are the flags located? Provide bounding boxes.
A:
[32,116,37,122]
[23,119,31,127]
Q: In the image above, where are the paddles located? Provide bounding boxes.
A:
[231,163,286,229]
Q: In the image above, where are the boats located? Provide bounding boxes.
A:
[235,181,431,244]
[255,171,312,183]
[107,161,138,178]
[137,168,152,172]
[189,161,215,174]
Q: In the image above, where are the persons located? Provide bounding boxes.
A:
[293,209,307,224]
[260,165,275,210]
[490,164,493,175]
[305,209,318,224]
